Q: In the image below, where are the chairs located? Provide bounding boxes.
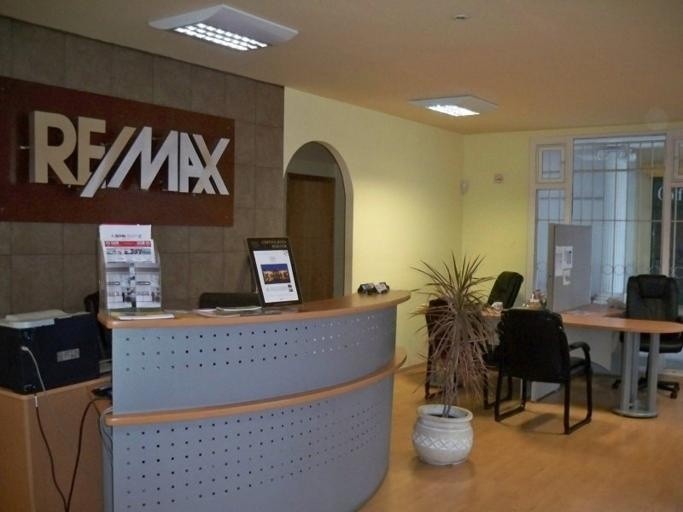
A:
[615,274,683,400]
[423,269,595,435]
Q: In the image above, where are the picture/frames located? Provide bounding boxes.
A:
[246,235,303,311]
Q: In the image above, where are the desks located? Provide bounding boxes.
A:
[556,299,682,420]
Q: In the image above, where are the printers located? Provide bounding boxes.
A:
[0,308,100,395]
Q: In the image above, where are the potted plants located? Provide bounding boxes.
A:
[409,250,504,469]
